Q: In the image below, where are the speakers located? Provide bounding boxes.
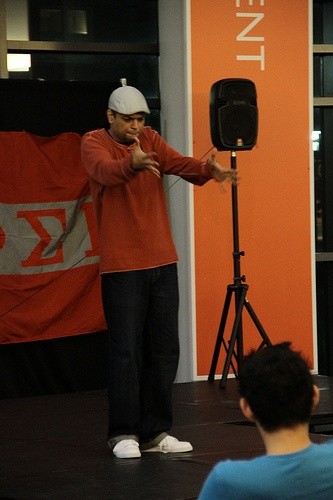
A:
[209,77,259,151]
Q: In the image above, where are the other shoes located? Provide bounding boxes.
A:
[112,439,140,458]
[140,435,192,453]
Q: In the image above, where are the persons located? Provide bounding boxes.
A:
[79,85,239,459]
[198,340,333,500]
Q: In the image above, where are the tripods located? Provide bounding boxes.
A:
[207,150,273,390]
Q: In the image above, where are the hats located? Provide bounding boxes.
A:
[108,78,150,114]
